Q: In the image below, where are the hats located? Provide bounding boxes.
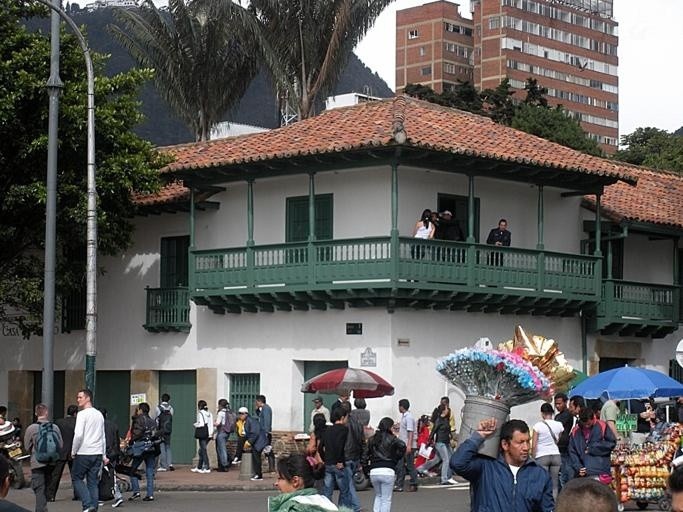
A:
[312,394,323,401]
[239,407,248,413]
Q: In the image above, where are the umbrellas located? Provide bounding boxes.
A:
[301,367,395,398]
[569,364,683,414]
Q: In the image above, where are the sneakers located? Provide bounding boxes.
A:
[392,470,458,492]
[80,457,275,512]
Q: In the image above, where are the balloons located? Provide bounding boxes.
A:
[496,324,579,396]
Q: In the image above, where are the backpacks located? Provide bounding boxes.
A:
[158,405,173,434]
[219,410,235,433]
[35,420,62,463]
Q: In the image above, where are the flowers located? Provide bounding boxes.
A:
[435,347,557,408]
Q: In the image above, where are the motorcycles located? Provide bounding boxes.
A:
[348,465,372,492]
[0,419,25,491]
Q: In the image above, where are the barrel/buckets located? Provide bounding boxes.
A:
[458,394,511,459]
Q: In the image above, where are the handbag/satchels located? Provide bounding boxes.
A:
[195,423,208,439]
[132,437,156,456]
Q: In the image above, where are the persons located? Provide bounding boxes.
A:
[416,397,459,485]
[267,450,354,512]
[232,407,269,481]
[362,417,407,512]
[97,403,162,507]
[214,399,230,472]
[0,405,33,512]
[486,219,511,287]
[393,399,418,492]
[23,404,64,512]
[71,389,110,512]
[155,393,175,472]
[450,417,555,512]
[50,404,81,502]
[255,395,276,474]
[407,209,467,284]
[532,393,683,512]
[305,394,370,512]
[190,400,214,473]
